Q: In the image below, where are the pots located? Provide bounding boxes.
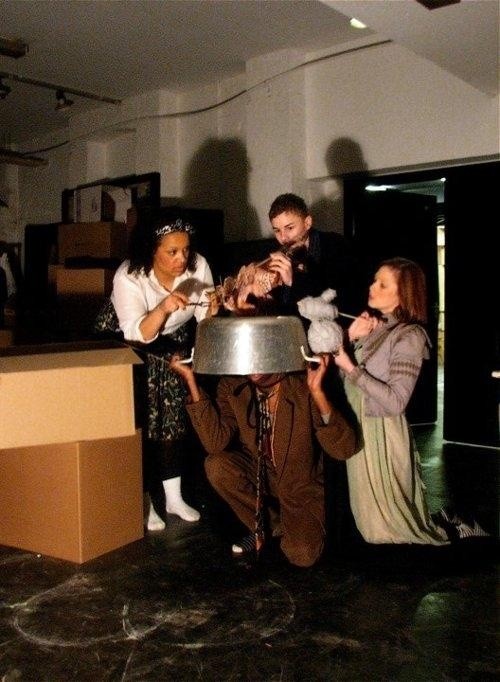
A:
[173,314,325,378]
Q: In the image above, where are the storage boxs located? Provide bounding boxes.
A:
[56,220,125,296]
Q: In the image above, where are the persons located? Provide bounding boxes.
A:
[95,212,219,535]
[333,255,489,549]
[169,345,357,569]
[254,191,376,313]
